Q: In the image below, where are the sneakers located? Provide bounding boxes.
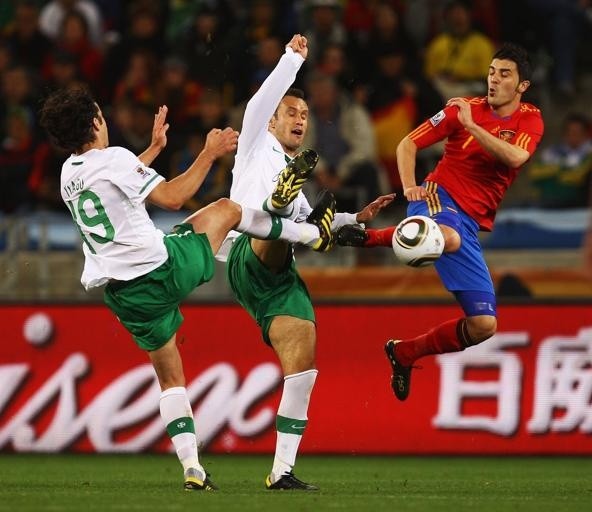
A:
[305,190,338,252]
[184,472,220,492]
[385,338,414,400]
[272,148,319,209]
[335,222,370,246]
[264,472,320,492]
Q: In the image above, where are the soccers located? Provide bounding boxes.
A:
[392,215,445,268]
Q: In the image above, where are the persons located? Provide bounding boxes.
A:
[40,33,336,492]
[0,0,592,210]
[215,34,397,491]
[336,46,545,400]
